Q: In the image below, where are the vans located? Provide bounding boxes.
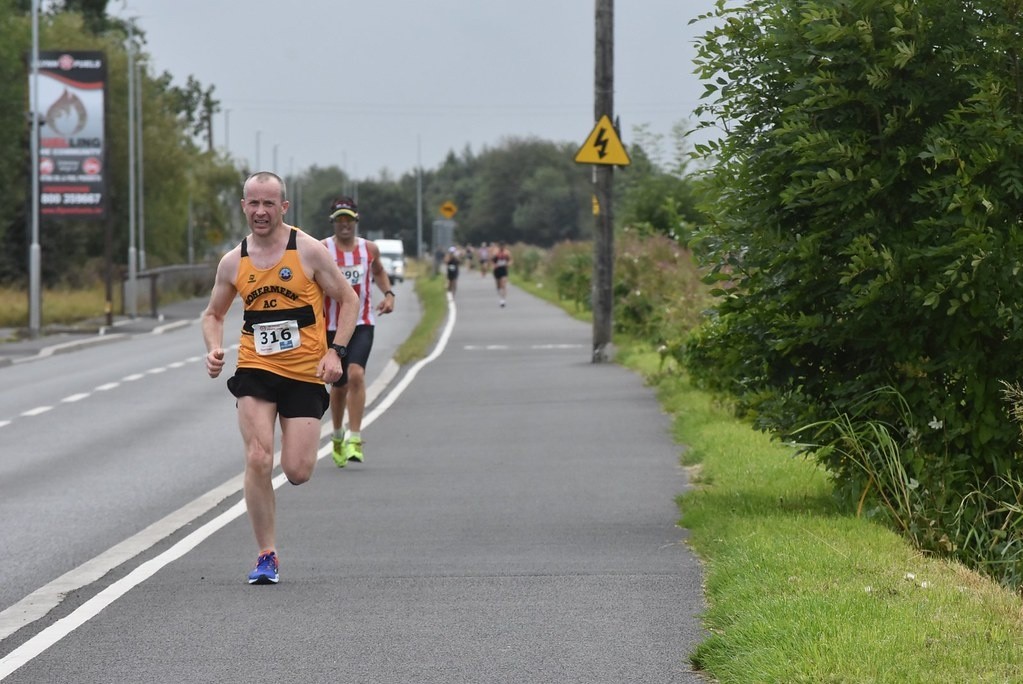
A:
[367,238,410,284]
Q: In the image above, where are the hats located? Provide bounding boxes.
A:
[329,196,359,222]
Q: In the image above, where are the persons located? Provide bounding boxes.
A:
[444,247,460,299]
[465,242,474,272]
[491,241,513,307]
[200,171,360,583]
[316,201,395,468]
[477,242,497,274]
[435,245,444,264]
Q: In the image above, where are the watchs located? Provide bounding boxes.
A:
[329,344,347,357]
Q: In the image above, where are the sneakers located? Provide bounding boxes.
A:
[330,429,348,468]
[248,550,279,585]
[346,436,365,463]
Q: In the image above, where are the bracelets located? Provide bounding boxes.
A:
[385,291,395,296]
